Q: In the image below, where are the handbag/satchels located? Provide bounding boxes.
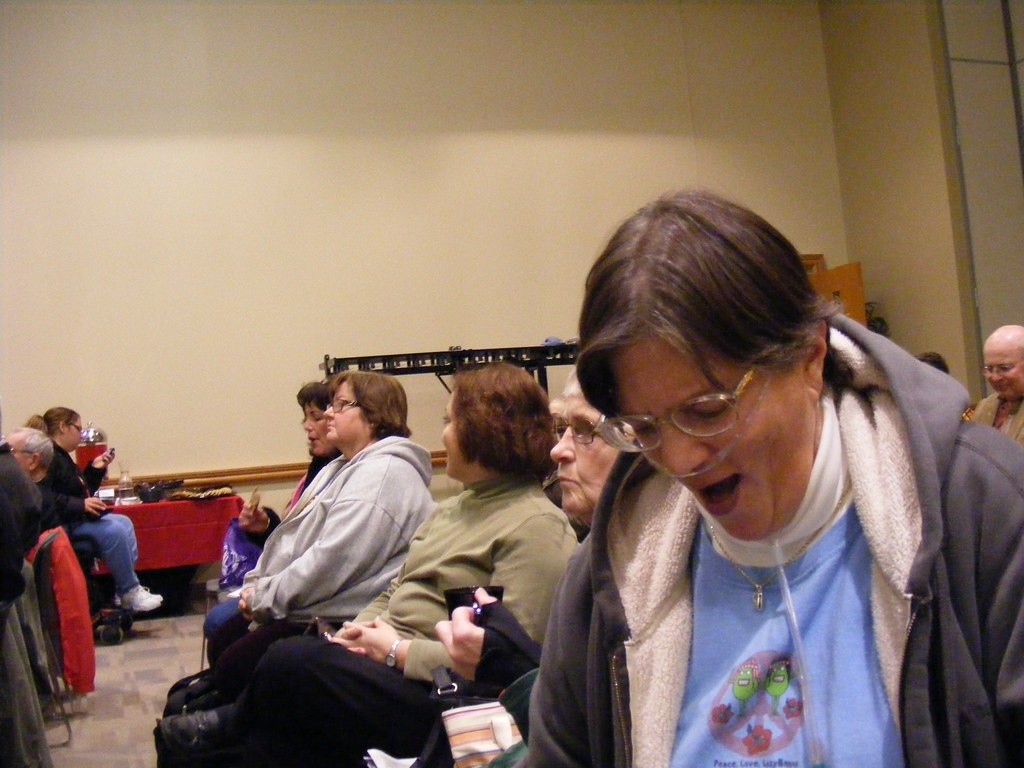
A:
[409,664,503,768]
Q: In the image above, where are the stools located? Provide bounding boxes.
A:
[200,578,220,671]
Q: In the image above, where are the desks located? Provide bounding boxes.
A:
[71,496,245,575]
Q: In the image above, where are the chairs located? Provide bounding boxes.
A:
[28,527,72,744]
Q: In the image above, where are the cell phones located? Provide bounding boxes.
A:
[102,448,115,462]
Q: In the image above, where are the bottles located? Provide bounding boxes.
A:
[117,459,137,506]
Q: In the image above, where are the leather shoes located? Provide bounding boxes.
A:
[159,706,242,752]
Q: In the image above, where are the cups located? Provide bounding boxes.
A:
[444,586,505,621]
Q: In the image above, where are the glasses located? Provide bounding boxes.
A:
[548,415,599,447]
[982,362,1019,376]
[591,368,761,451]
[69,422,82,432]
[10,447,34,457]
[326,399,363,415]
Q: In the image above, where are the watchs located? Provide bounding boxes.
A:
[384,639,401,667]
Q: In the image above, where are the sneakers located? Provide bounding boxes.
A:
[114,585,164,612]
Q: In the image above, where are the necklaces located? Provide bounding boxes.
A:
[709,479,853,612]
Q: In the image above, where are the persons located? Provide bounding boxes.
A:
[5,427,60,533]
[201,382,343,639]
[516,191,1024,768]
[152,362,578,768]
[25,407,163,611]
[971,324,1024,440]
[433,371,636,768]
[0,437,42,629]
[207,371,434,685]
